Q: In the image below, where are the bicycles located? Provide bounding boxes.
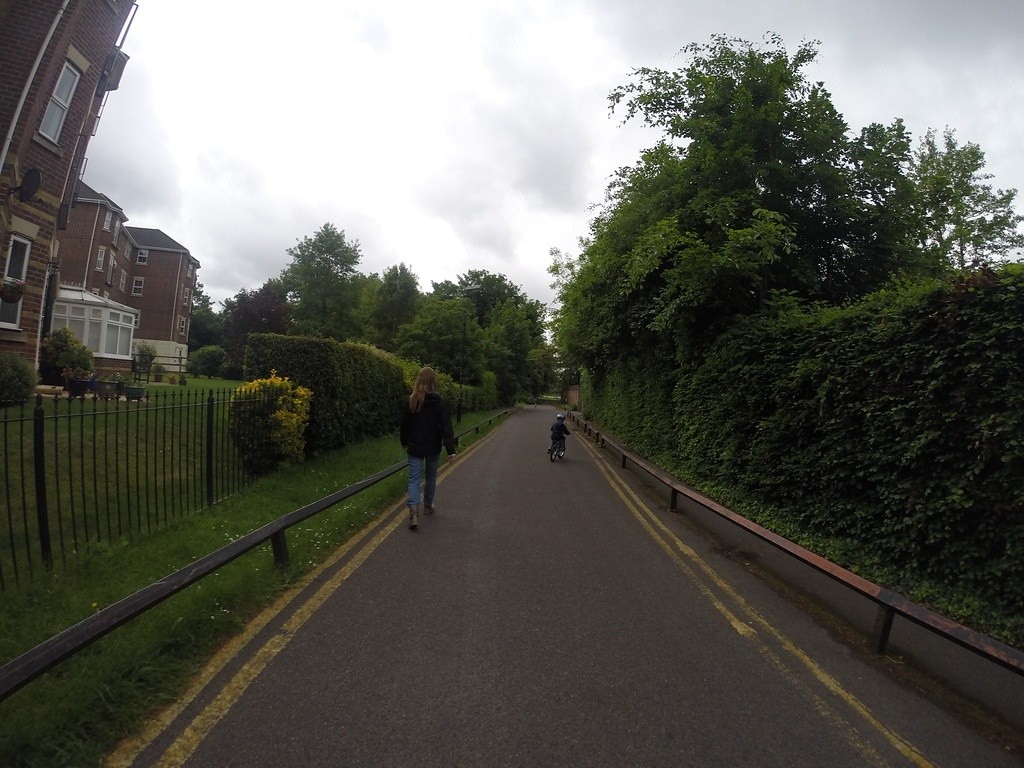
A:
[549,431,568,461]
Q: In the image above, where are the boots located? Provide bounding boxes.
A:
[424,505,434,514]
[408,505,419,528]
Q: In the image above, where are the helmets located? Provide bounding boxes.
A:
[557,413,566,418]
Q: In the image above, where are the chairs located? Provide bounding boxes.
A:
[132,355,150,384]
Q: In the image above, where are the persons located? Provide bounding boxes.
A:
[547,414,571,454]
[399,367,457,529]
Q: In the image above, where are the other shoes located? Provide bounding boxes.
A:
[560,452,564,456]
[547,446,551,454]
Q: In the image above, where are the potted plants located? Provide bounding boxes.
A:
[61,367,94,396]
[179,375,187,385]
[168,375,176,384]
[136,340,157,370]
[94,374,119,396]
[147,359,168,382]
[124,380,146,400]
[0,278,27,305]
[119,375,134,394]
